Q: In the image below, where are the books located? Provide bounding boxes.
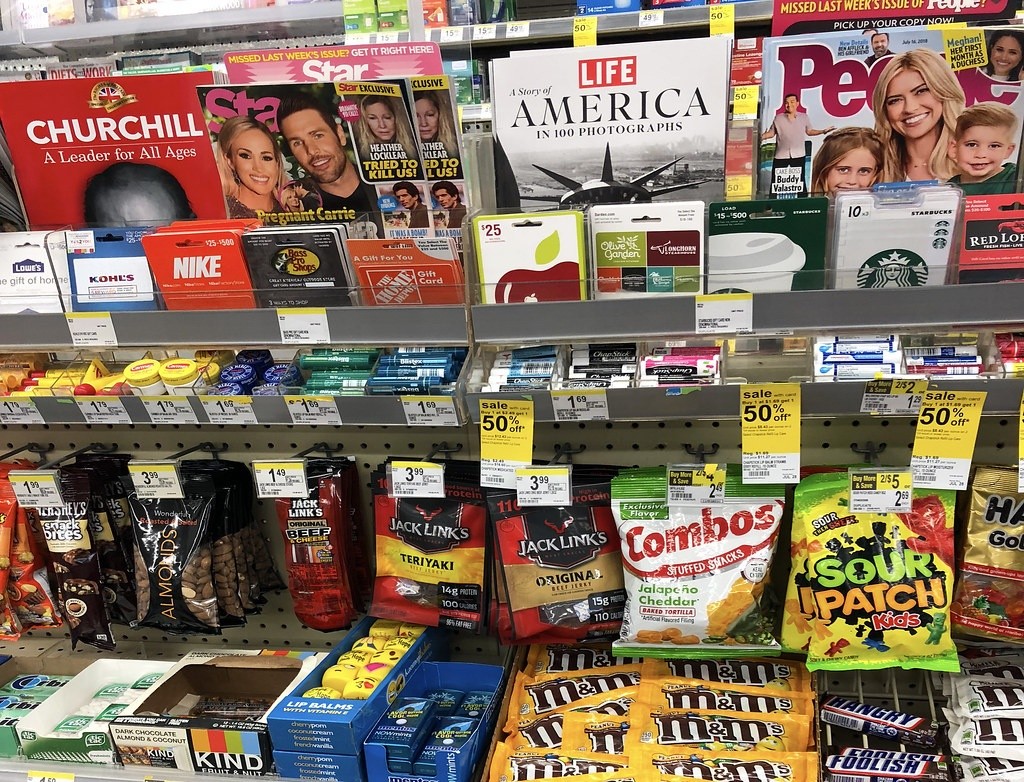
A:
[488,20,1024,199]
[1,70,470,275]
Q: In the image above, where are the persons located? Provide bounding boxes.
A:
[393,181,429,228]
[276,92,384,239]
[872,47,966,184]
[294,178,321,212]
[862,29,878,35]
[281,187,304,213]
[218,116,290,226]
[762,94,837,200]
[986,30,1024,81]
[354,95,417,160]
[83,161,197,226]
[413,91,458,159]
[432,181,467,228]
[811,126,885,198]
[864,33,897,68]
[944,101,1019,195]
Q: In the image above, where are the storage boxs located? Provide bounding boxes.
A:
[0,615,824,782]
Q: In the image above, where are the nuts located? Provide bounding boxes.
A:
[133,525,280,628]
